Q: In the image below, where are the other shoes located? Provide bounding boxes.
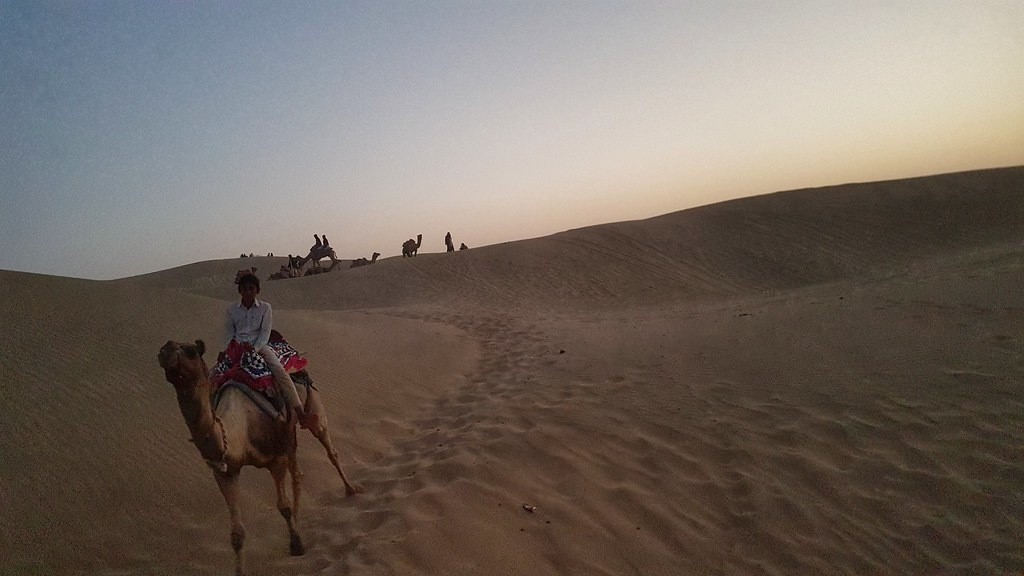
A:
[299,413,318,430]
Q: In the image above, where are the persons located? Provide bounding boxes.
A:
[444,231,469,252]
[313,234,328,250]
[186,272,324,445]
[279,254,298,278]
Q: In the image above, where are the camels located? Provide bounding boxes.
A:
[402,234,422,259]
[234,244,342,284]
[157,328,358,576]
[350,252,381,269]
